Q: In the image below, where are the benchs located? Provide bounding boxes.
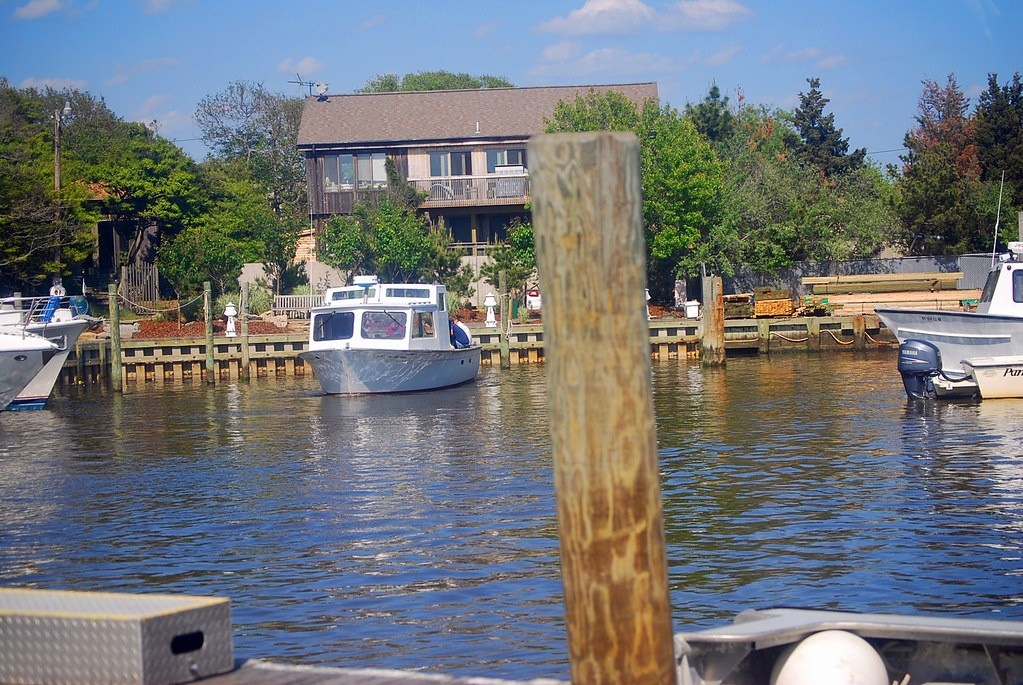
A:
[270,292,325,319]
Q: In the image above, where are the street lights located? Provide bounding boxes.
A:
[52,101,70,286]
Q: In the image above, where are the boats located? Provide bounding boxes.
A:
[297,275,483,395]
[873,241,1023,402]
[0,284,106,414]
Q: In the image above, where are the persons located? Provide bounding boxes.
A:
[448,314,472,349]
[387,319,404,337]
